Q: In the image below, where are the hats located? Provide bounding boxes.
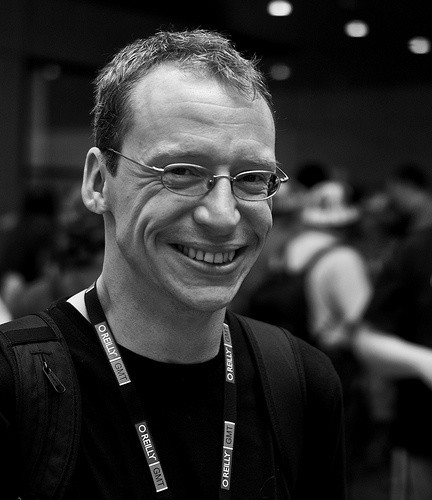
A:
[302,182,355,222]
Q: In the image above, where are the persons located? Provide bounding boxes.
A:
[0,30,346,500]
[0,160,432,500]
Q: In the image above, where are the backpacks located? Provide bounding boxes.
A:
[234,241,349,346]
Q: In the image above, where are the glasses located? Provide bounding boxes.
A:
[107,143,289,201]
